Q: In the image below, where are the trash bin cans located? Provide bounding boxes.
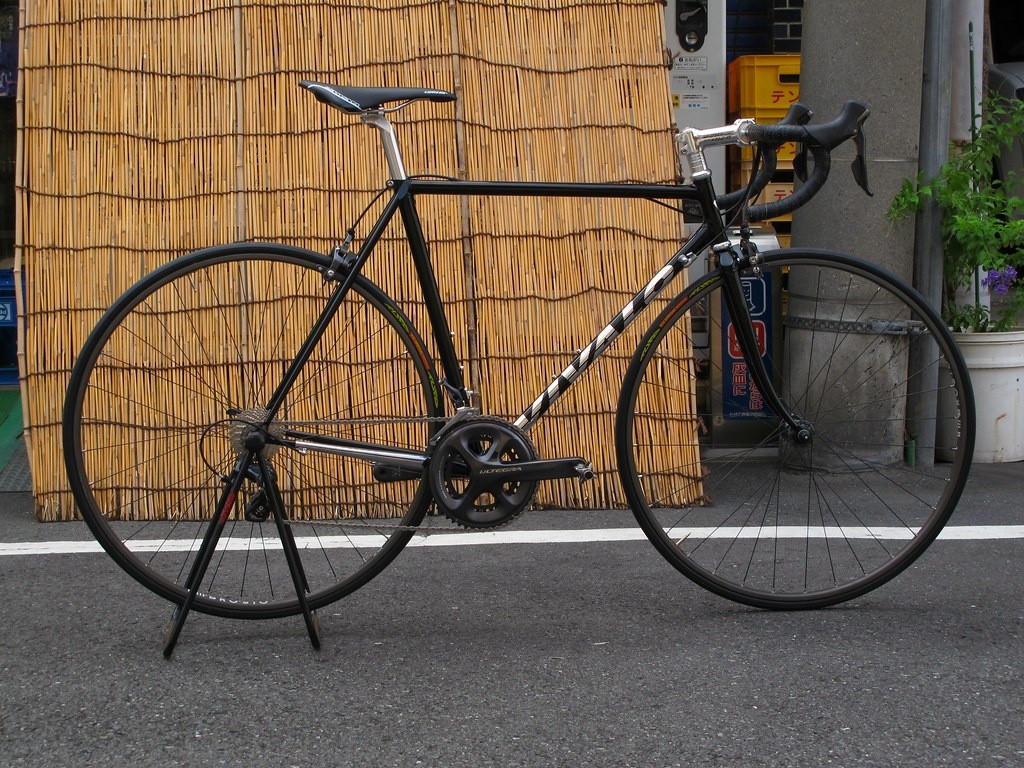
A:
[707,222,784,439]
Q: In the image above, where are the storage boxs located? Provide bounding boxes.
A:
[728,53,801,315]
[0,268,27,384]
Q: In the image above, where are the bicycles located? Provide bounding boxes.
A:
[61,77,978,617]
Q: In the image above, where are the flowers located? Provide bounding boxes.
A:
[982,265,1017,300]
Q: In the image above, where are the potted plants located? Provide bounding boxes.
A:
[884,91,1024,465]
